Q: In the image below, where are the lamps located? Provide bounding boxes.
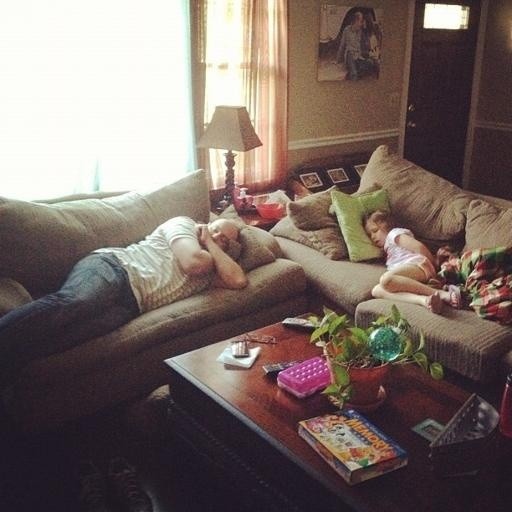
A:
[196,104,264,204]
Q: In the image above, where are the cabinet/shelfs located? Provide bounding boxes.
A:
[237,207,279,230]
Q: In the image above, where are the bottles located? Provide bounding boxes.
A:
[500,375,512,439]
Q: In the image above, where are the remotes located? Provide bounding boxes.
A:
[283,318,323,330]
[261,360,300,377]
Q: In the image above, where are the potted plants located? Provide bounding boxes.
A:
[310,298,446,415]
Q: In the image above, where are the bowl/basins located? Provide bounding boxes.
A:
[255,203,284,220]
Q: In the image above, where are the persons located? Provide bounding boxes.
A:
[362,208,461,315]
[0,215,249,355]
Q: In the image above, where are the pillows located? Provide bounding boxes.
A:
[285,184,348,260]
[266,215,317,250]
[328,185,395,263]
[218,203,276,271]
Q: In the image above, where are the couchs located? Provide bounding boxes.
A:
[0,167,311,436]
[269,141,511,400]
